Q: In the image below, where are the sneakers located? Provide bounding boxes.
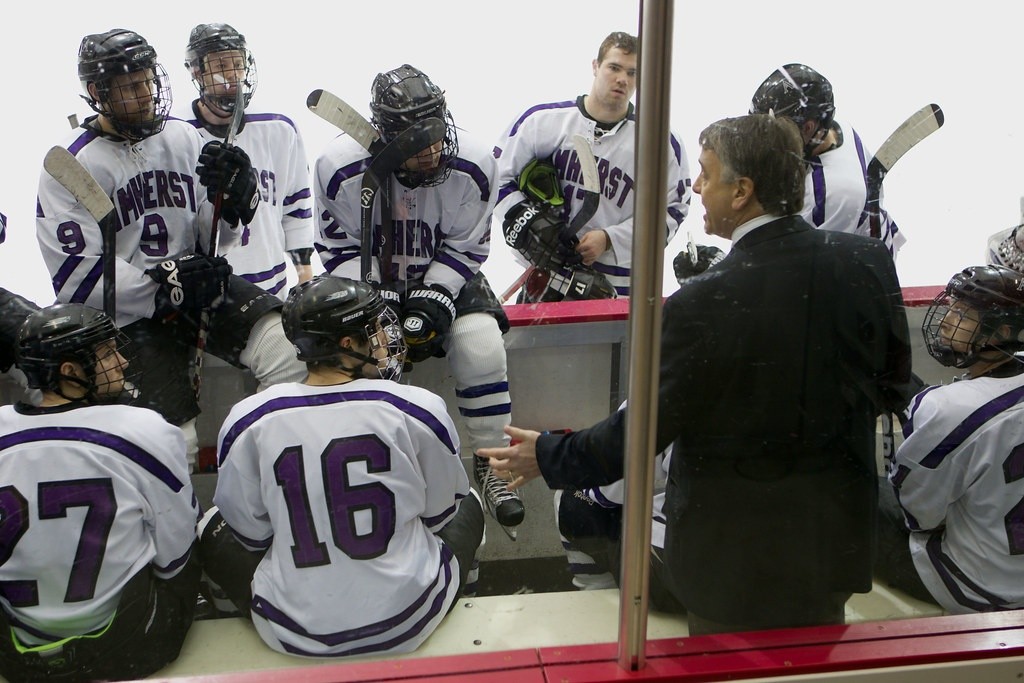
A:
[472,453,524,544]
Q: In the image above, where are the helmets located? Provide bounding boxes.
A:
[186,24,259,117]
[370,65,459,188]
[280,277,408,384]
[195,505,264,615]
[749,63,836,161]
[14,304,140,409]
[76,26,173,138]
[523,262,619,301]
[921,265,1024,368]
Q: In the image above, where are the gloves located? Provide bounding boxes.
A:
[370,281,406,330]
[195,141,261,227]
[403,283,457,362]
[144,252,231,312]
[674,244,729,286]
[506,202,585,270]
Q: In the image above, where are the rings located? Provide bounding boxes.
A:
[509,472,512,478]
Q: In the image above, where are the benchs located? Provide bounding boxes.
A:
[140,572,961,681]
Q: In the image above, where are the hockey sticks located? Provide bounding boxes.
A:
[190,78,244,430]
[306,89,393,284]
[361,117,446,286]
[866,103,944,480]
[498,134,600,305]
[43,145,116,324]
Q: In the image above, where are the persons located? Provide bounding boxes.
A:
[673,64,907,288]
[183,22,316,367]
[474,114,914,636]
[0,302,205,683]
[553,397,686,615]
[872,264,1024,613]
[313,63,525,528]
[197,277,484,657]
[36,29,309,477]
[494,32,693,305]
